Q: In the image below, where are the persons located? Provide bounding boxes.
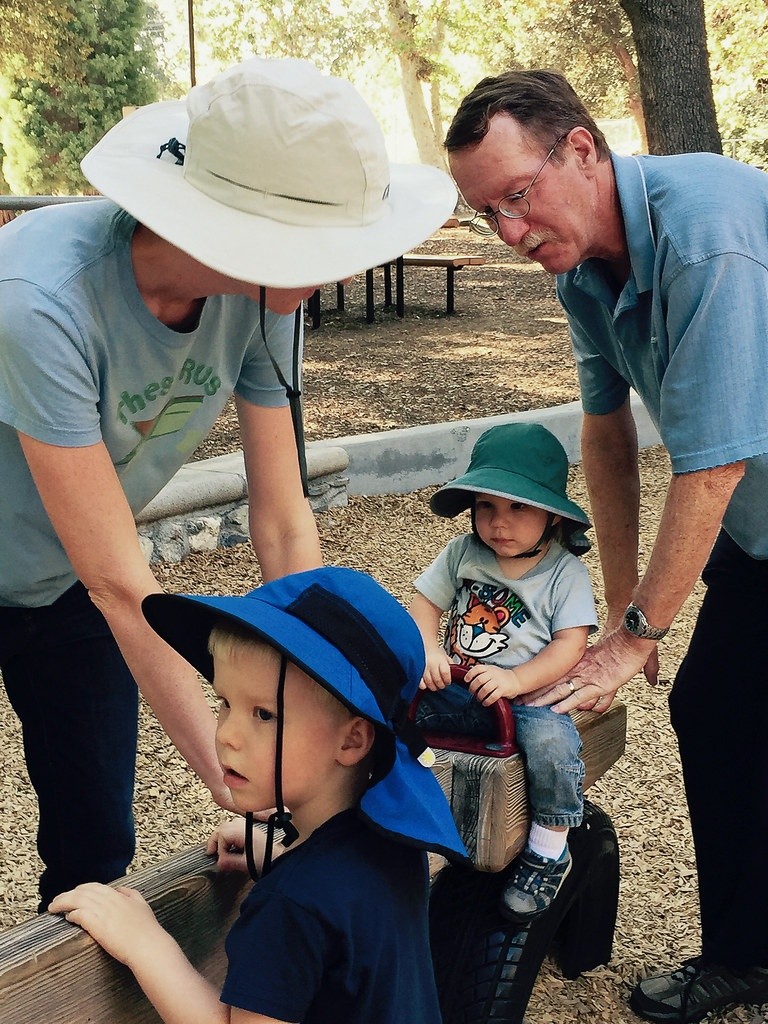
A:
[408,422,593,918]
[443,71,767,1024]
[0,59,460,914]
[46,566,470,1024]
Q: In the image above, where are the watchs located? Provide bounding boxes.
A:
[623,601,670,640]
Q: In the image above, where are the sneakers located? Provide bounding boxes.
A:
[631,951,768,1024]
[503,845,574,920]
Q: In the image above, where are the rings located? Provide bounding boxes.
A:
[566,680,575,694]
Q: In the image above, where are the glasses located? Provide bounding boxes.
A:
[466,134,570,238]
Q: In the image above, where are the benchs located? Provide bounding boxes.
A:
[389,254,485,318]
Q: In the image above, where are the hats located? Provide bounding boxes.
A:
[428,421,594,556]
[80,59,461,289]
[142,567,476,869]
